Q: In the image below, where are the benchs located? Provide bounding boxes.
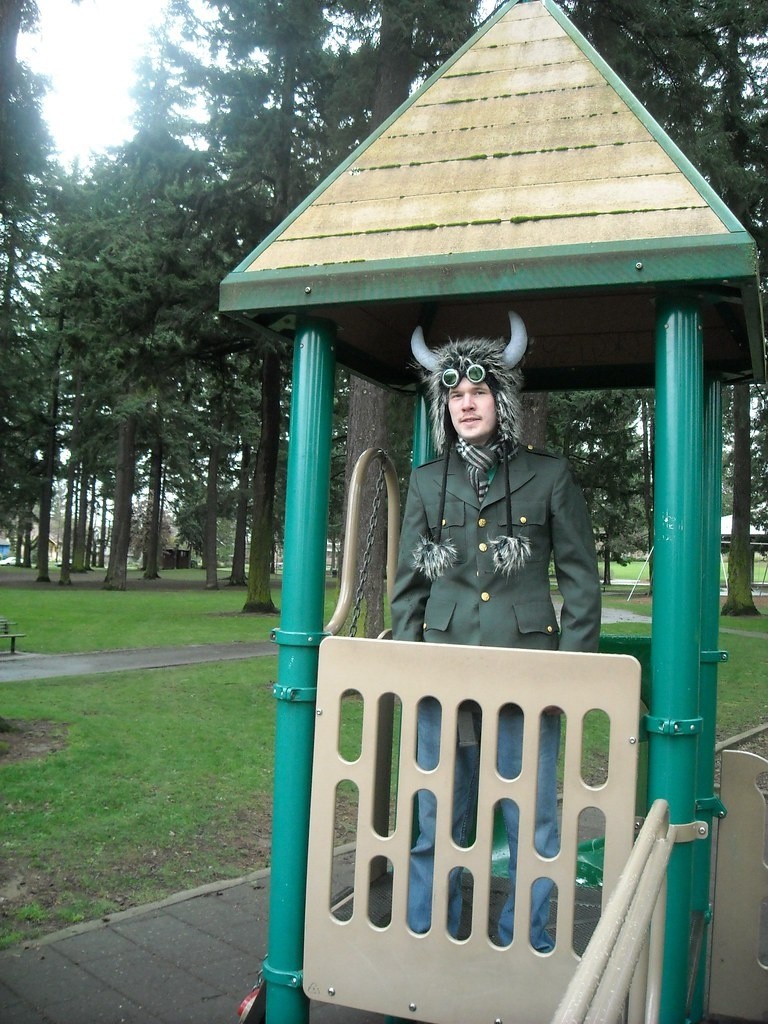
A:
[0,633,27,638]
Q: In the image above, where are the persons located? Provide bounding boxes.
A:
[393,337,602,954]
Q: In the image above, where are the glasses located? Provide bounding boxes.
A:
[442,364,486,388]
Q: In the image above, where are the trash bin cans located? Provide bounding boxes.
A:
[177,549,189,569]
[162,548,176,569]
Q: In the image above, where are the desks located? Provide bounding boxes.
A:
[0,616,26,655]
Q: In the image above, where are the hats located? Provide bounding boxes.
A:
[406,310,531,582]
